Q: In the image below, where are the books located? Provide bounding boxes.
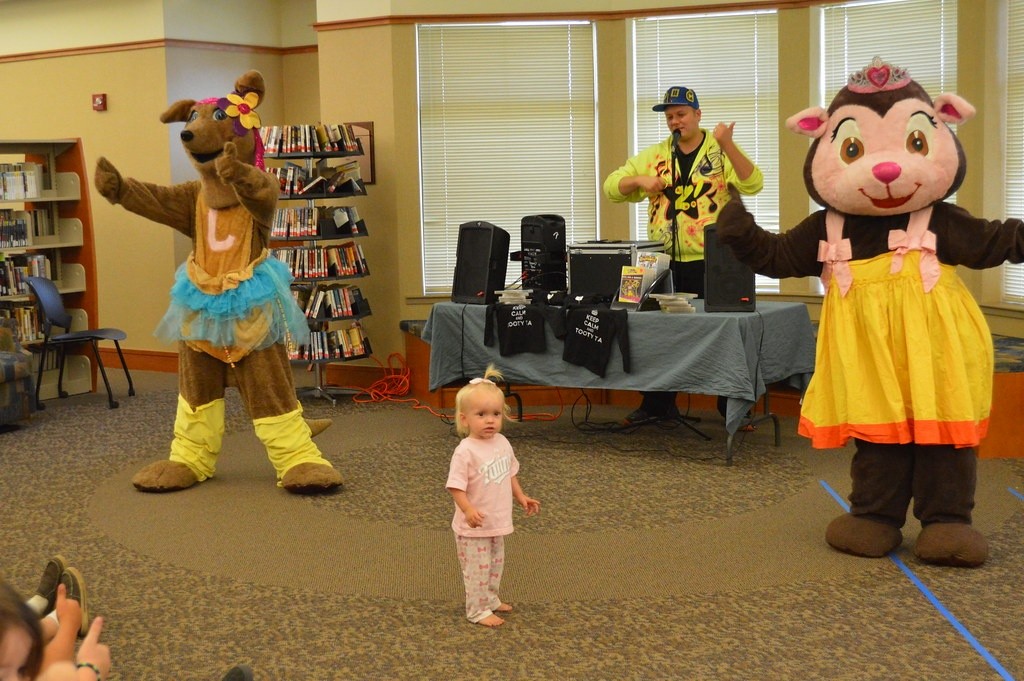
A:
[291,283,371,319]
[494,288,533,304]
[270,205,362,238]
[285,322,373,361]
[619,251,699,313]
[259,123,358,153]
[0,207,59,372]
[0,162,47,199]
[263,157,366,194]
[269,239,371,278]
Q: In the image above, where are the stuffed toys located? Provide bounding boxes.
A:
[95,69,343,496]
[715,55,1023,569]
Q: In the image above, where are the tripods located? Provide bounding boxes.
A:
[610,147,711,440]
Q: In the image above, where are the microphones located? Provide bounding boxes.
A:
[671,129,681,148]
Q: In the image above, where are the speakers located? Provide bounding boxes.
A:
[703,223,756,311]
[452,221,511,304]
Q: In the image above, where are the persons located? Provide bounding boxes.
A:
[445,365,541,628]
[601,87,765,430]
[1,556,111,681]
[221,667,252,681]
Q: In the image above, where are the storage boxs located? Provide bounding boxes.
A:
[567,240,666,311]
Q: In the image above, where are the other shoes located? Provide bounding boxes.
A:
[35,555,68,616]
[724,415,755,430]
[61,566,88,637]
[223,664,253,681]
[624,399,661,425]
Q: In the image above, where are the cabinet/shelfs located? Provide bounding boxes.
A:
[262,137,373,407]
[0,137,99,401]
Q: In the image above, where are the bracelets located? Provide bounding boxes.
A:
[77,662,101,681]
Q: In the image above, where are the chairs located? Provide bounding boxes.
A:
[21,276,135,410]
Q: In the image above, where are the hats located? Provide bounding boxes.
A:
[653,87,699,112]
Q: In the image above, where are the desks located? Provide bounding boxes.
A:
[420,299,816,469]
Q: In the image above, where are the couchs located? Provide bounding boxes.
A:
[0,317,37,427]
